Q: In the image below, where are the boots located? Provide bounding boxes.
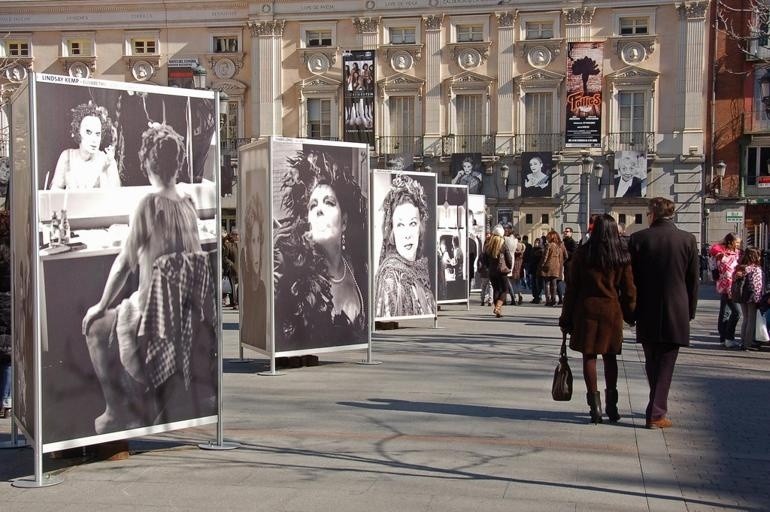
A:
[494,298,504,317]
[605,388,620,423]
[587,391,603,425]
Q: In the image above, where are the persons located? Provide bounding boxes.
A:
[375,175,436,316]
[273,151,368,352]
[621,198,699,429]
[478,214,598,318]
[521,156,551,196]
[49,103,122,189]
[224,228,239,310]
[614,157,641,198]
[708,233,742,348]
[560,213,637,425]
[82,124,203,435]
[344,62,373,91]
[451,157,483,194]
[731,246,767,352]
[440,236,464,280]
[222,235,229,305]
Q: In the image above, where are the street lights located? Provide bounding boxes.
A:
[580,150,595,235]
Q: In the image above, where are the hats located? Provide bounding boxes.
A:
[493,227,504,236]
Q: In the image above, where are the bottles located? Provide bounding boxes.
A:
[59,210,70,244]
[50,211,61,248]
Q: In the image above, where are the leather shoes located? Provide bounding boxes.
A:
[646,418,672,429]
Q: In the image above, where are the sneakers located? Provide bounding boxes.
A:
[721,340,760,352]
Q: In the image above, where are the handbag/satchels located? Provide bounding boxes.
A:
[552,333,573,401]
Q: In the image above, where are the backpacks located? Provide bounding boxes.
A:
[732,271,755,304]
[709,256,720,280]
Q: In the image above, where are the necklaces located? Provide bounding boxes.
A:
[326,253,364,315]
